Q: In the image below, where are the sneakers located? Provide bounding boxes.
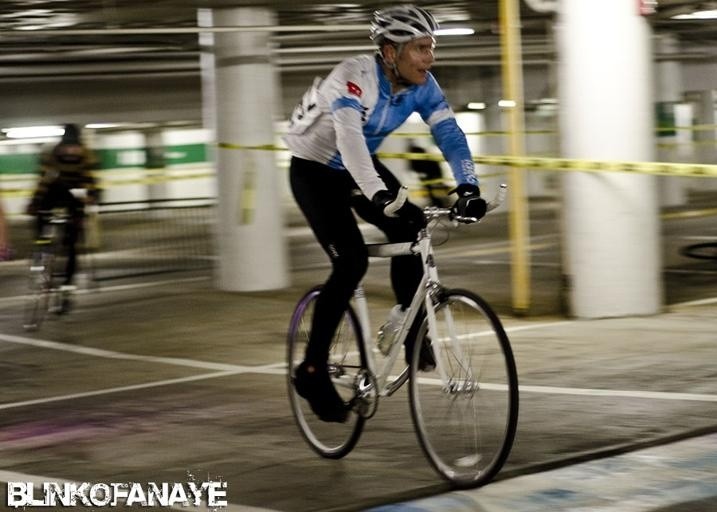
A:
[294,362,347,422]
[399,313,436,373]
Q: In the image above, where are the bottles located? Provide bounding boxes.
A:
[377,304,408,356]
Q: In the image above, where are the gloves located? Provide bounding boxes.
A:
[371,188,425,225]
[449,185,486,223]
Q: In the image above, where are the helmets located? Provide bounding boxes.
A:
[369,5,440,40]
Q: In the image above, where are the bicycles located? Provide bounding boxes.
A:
[21,210,74,331]
[284,183,520,488]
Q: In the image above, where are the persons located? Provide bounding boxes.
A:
[25,124,104,314]
[279,3,486,421]
